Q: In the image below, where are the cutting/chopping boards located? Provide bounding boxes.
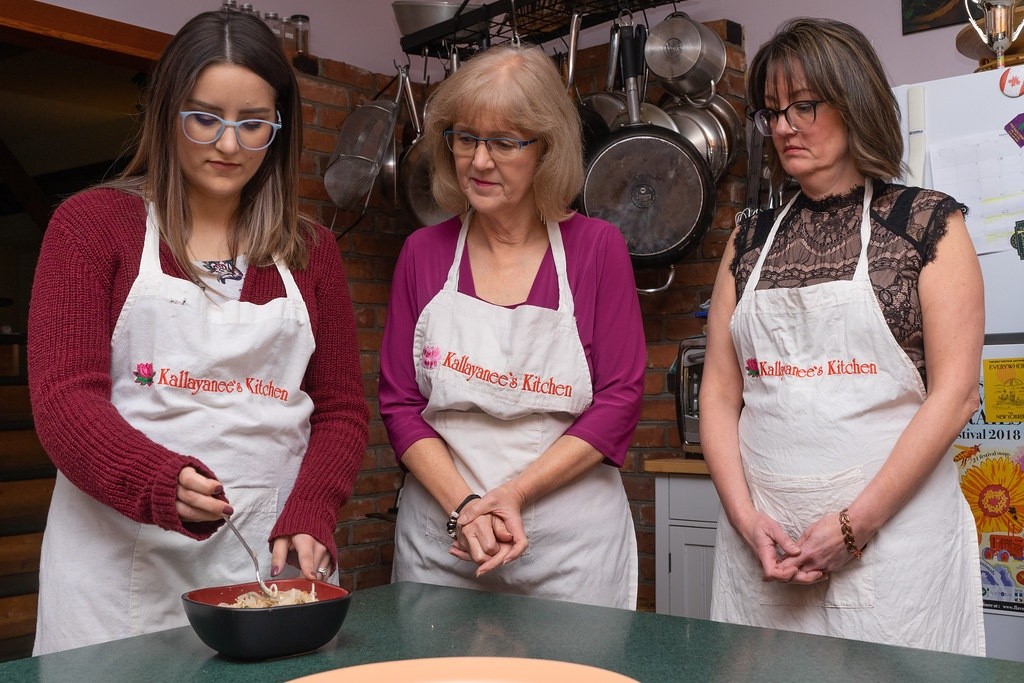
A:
[282,655,643,683]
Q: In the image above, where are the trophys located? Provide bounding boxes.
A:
[964,0,1024,69]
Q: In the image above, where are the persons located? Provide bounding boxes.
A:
[376,46,647,611]
[26,10,371,656]
[699,16,986,659]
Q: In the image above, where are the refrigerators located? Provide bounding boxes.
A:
[888,64,1024,662]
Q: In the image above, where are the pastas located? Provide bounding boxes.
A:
[217,583,319,608]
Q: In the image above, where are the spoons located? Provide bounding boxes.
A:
[224,515,275,597]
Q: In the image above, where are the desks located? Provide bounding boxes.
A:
[1,580,1024,683]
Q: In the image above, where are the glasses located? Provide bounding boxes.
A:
[443,130,542,161]
[179,108,282,150]
[753,101,827,135]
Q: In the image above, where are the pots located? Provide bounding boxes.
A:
[546,8,743,299]
[376,47,464,231]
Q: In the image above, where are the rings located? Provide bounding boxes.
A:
[317,567,328,576]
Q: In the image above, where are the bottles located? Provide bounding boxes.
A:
[220,0,310,67]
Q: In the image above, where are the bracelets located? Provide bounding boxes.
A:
[447,494,482,540]
[839,508,863,558]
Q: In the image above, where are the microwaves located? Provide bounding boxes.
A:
[667,333,705,461]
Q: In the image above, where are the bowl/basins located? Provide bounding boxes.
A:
[391,1,494,41]
[181,578,352,662]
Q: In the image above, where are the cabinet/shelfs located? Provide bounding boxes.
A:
[643,460,1023,661]
